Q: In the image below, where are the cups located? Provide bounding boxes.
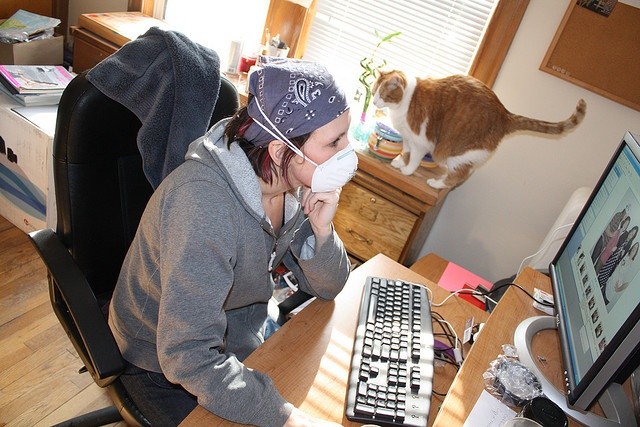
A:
[513,396,568,427]
[502,417,543,427]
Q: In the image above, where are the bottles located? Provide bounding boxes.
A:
[367,121,403,162]
[226,37,242,75]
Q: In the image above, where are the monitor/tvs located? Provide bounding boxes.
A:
[513,130,639,427]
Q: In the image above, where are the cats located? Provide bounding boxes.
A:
[370,64,588,190]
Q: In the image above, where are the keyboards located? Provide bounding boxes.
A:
[346,276,435,427]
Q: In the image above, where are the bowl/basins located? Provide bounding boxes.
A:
[494,361,542,408]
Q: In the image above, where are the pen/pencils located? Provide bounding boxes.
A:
[265,28,270,47]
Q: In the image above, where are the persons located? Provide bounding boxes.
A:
[106,54,358,427]
[598,226,638,292]
[591,203,631,265]
[601,242,639,305]
[594,216,631,276]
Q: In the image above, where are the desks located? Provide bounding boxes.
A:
[407,252,492,311]
[178,251,640,427]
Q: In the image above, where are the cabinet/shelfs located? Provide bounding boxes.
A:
[70,13,448,269]
[0,79,62,236]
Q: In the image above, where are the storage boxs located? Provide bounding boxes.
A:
[0,19,64,65]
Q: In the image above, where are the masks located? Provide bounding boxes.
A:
[249,98,358,193]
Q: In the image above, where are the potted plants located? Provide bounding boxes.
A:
[345,28,402,153]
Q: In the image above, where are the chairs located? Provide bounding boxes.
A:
[28,70,240,426]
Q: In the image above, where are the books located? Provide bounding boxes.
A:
[0,9,62,43]
[0,65,75,108]
